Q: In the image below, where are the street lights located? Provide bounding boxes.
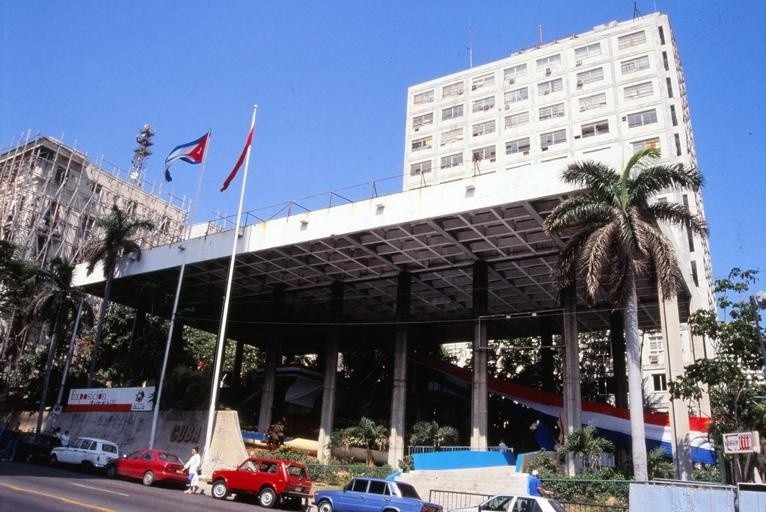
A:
[37,266,86,430]
[750,290,766,375]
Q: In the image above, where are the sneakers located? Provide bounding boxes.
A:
[184,489,195,493]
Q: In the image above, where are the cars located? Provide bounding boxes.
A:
[0,431,192,488]
[314,476,445,512]
[452,493,568,512]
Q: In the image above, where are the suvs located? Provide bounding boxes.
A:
[210,456,313,511]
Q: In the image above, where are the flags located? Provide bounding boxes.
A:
[218,123,256,193]
[163,130,211,186]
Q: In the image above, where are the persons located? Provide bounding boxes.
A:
[53,426,63,440]
[180,446,204,495]
[0,421,14,459]
[2,421,22,463]
[529,469,547,497]
[43,427,57,438]
[59,430,71,447]
